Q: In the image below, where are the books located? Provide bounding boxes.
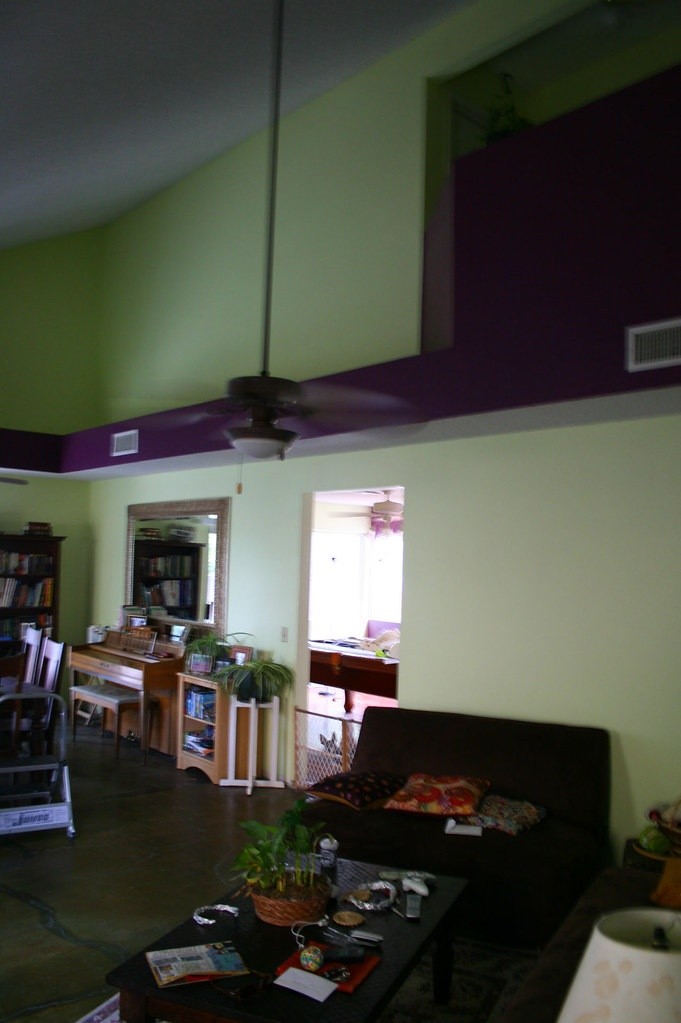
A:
[0,550,54,638]
[185,727,213,755]
[186,688,216,719]
[134,555,192,617]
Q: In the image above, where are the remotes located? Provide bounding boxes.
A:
[378,870,437,882]
[405,895,423,919]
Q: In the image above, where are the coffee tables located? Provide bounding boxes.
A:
[106,850,473,1023]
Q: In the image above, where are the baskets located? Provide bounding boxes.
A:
[247,868,335,931]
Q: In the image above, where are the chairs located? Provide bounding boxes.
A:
[0,626,68,799]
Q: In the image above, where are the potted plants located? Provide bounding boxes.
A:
[228,797,337,929]
[209,658,297,704]
[185,626,249,679]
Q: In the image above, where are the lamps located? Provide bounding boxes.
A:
[207,0,314,461]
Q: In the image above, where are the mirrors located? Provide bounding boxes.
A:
[124,496,232,627]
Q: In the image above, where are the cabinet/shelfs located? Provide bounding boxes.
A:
[175,671,264,786]
[0,533,67,679]
[132,536,202,623]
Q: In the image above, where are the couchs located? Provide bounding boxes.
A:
[294,707,611,914]
[496,869,681,1023]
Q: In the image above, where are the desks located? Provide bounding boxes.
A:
[0,676,56,805]
[67,634,186,765]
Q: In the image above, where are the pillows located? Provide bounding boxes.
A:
[459,795,545,835]
[384,773,491,815]
[305,768,406,812]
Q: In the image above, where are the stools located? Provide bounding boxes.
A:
[70,684,158,767]
